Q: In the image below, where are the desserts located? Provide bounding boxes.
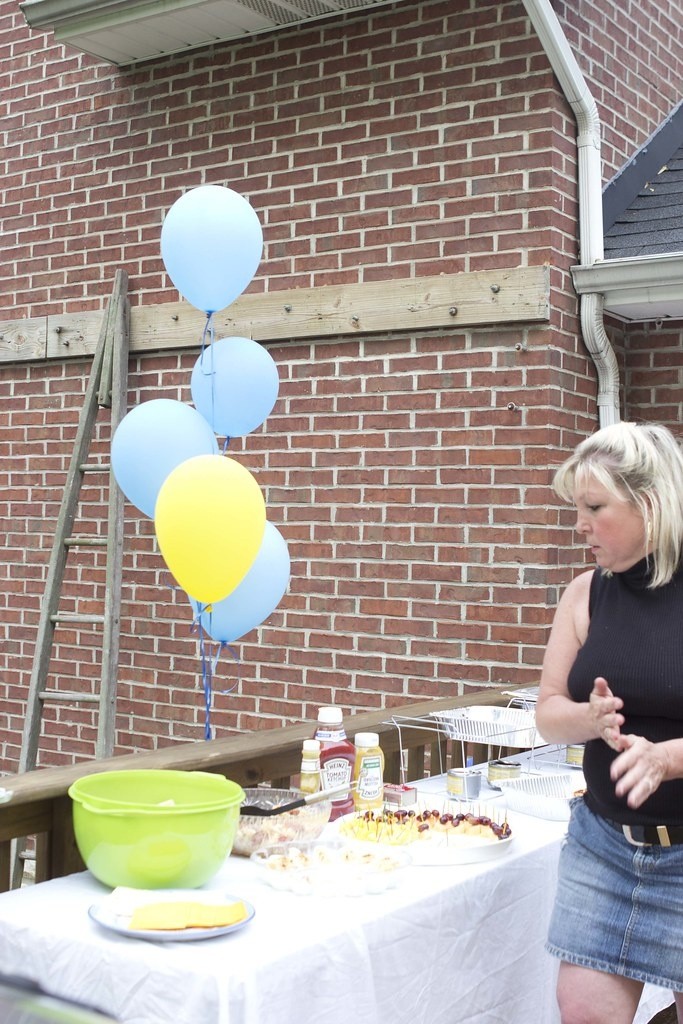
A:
[336,810,512,849]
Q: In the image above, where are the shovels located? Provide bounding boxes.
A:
[239,781,358,817]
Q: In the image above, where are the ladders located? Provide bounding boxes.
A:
[8,268,128,892]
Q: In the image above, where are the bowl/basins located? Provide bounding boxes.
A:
[234,788,332,857]
[67,769,247,890]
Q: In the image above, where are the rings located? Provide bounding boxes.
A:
[644,777,653,791]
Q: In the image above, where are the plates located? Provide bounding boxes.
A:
[251,841,411,897]
[90,893,256,942]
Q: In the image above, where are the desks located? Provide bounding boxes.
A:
[0,743,676,1024]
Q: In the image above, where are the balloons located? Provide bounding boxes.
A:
[111,398,219,521]
[188,519,290,642]
[154,454,267,613]
[160,184,264,318]
[192,337,280,437]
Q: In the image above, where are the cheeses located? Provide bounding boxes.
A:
[125,901,246,929]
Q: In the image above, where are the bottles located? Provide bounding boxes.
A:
[353,732,385,810]
[566,744,585,765]
[447,767,481,800]
[315,706,356,822]
[488,760,522,791]
[300,740,322,799]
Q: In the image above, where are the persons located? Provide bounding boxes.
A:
[535,421,683,1024]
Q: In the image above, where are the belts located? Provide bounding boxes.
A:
[603,816,683,847]
